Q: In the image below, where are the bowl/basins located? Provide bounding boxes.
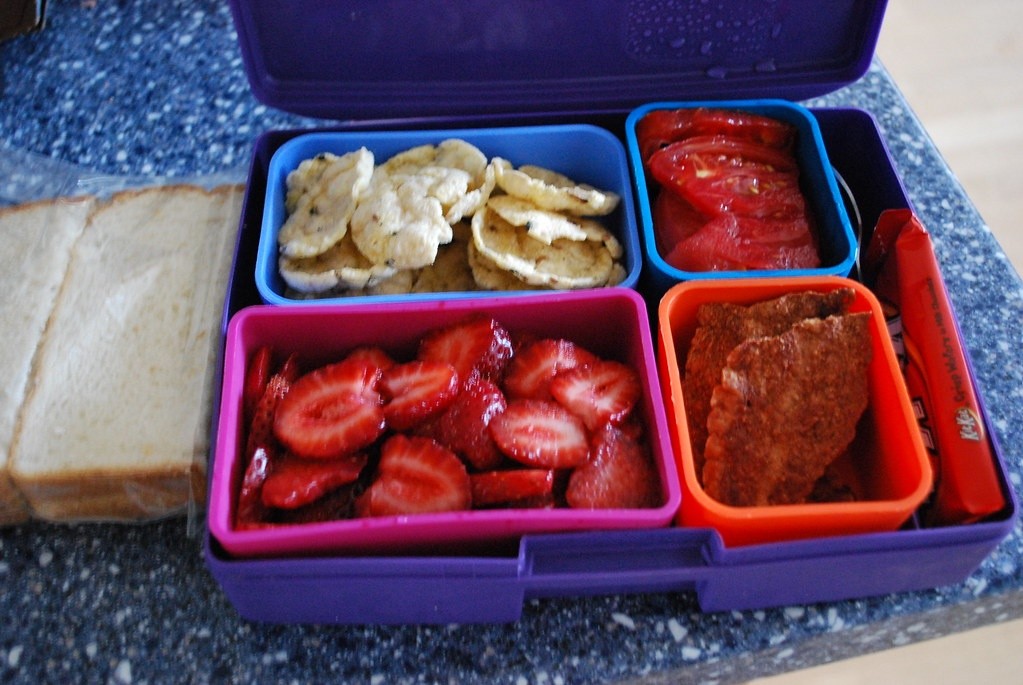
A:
[658,276,932,549]
[208,287,681,554]
[254,124,642,309]
[625,99,858,280]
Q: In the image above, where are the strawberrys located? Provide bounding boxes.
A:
[232,312,659,527]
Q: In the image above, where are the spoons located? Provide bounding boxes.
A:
[831,161,863,282]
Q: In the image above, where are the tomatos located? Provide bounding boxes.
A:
[638,108,821,272]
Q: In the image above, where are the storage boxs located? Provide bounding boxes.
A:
[203,0,1017,630]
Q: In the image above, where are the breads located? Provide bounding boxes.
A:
[0,183,244,524]
